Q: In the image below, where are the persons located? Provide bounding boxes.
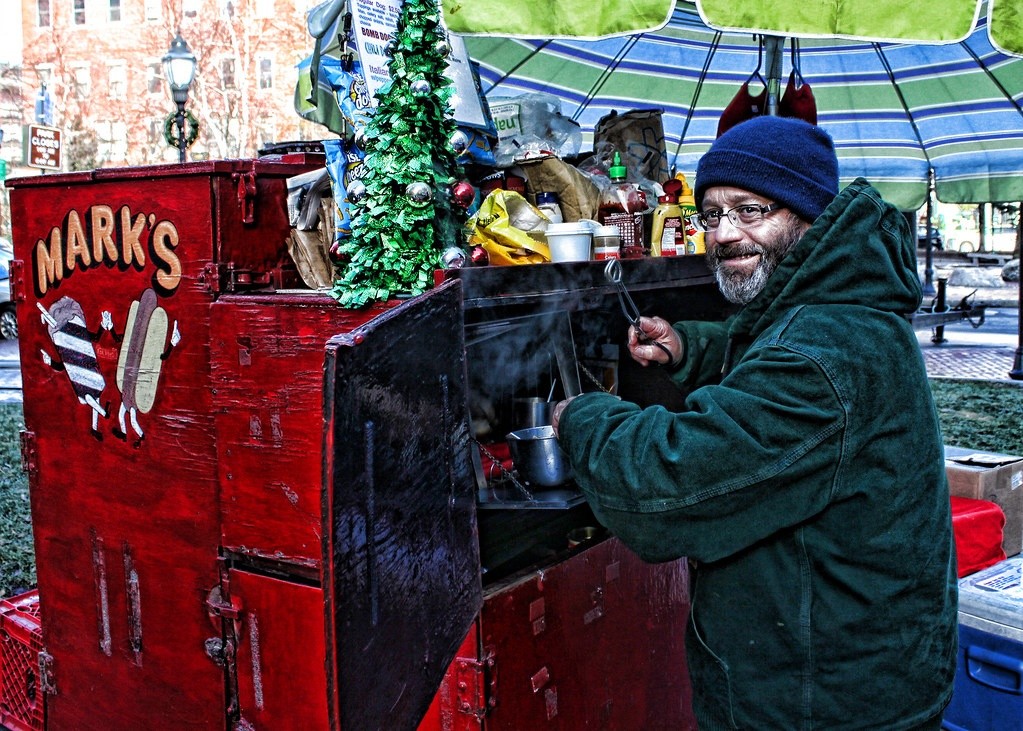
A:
[550,116,957,731]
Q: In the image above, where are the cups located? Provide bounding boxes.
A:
[544,221,594,263]
[512,396,557,431]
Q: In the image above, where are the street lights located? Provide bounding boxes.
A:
[159,32,197,163]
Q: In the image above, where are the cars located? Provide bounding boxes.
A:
[0,247,19,342]
[918,224,943,252]
[1002,258,1020,283]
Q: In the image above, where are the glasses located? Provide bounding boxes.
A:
[690,203,786,233]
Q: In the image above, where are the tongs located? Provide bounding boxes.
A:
[604,259,673,372]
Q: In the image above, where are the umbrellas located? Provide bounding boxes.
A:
[294,0,1023,213]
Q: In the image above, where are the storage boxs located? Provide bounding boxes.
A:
[942,554,1023,731]
[945,453,1023,557]
[949,495,1007,578]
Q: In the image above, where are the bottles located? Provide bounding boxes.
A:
[650,178,688,257]
[597,152,644,259]
[674,172,706,255]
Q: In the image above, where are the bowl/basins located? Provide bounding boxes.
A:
[505,425,572,488]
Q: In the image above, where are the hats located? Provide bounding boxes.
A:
[696,116,839,223]
